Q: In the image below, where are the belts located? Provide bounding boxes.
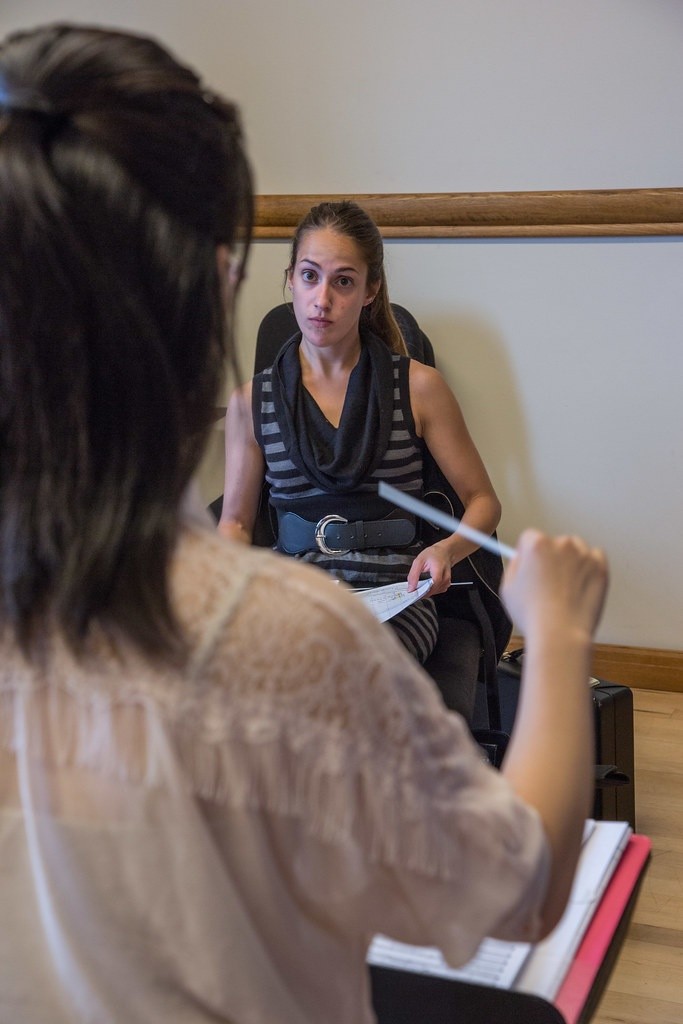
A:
[274,508,422,556]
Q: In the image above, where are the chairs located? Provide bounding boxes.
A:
[208,302,509,770]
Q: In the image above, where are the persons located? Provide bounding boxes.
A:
[217,201,502,669]
[0,23,610,1024]
